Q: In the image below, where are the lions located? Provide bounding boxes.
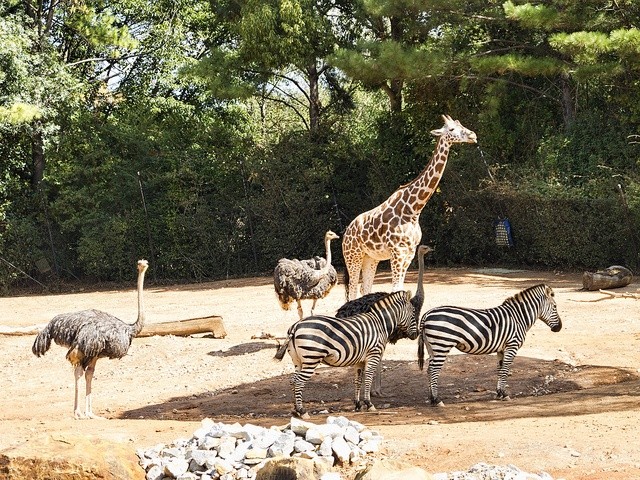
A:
[272,291,420,421]
[415,283,562,410]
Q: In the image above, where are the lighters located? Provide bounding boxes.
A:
[337,112,478,305]
